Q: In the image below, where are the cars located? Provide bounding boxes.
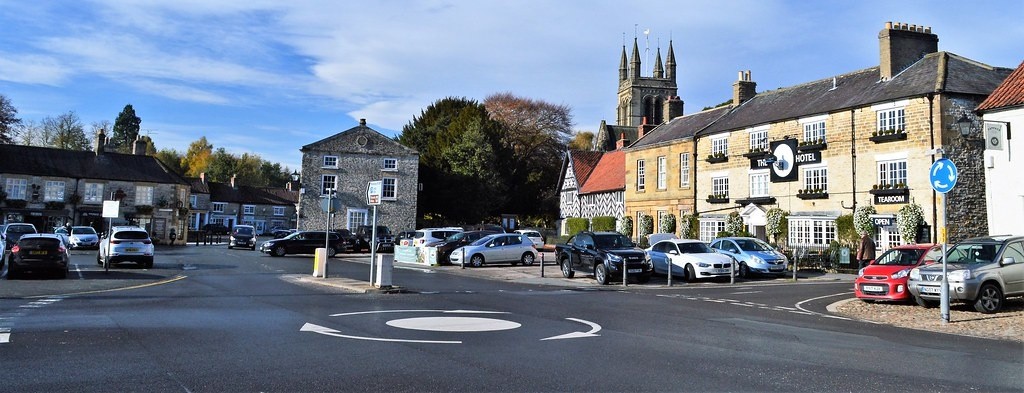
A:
[54,225,100,250]
[226,225,259,251]
[425,229,505,265]
[449,233,539,267]
[554,231,653,285]
[395,231,415,247]
[907,235,1023,314]
[513,230,545,250]
[640,233,740,283]
[0,231,6,265]
[708,236,789,276]
[326,228,361,253]
[854,243,966,303]
[2,223,38,249]
[270,225,298,240]
[8,234,70,279]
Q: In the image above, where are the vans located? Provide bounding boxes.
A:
[413,228,464,247]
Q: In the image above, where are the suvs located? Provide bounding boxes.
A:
[355,225,396,252]
[259,231,347,258]
[97,226,154,269]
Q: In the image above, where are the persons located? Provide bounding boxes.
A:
[62,222,72,235]
[857,231,875,276]
[97,231,108,242]
